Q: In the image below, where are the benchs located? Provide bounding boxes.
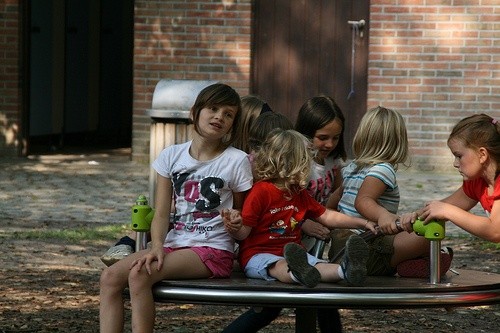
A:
[124,269,500,333]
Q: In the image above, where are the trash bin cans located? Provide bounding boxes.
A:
[148,77,230,216]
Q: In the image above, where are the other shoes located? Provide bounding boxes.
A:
[100,243,132,266]
[340,233,369,285]
[398,245,452,281]
[283,242,321,287]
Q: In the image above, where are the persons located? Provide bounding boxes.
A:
[100,84,255,333]
[101,96,378,288]
[400,113,500,243]
[324,105,454,279]
